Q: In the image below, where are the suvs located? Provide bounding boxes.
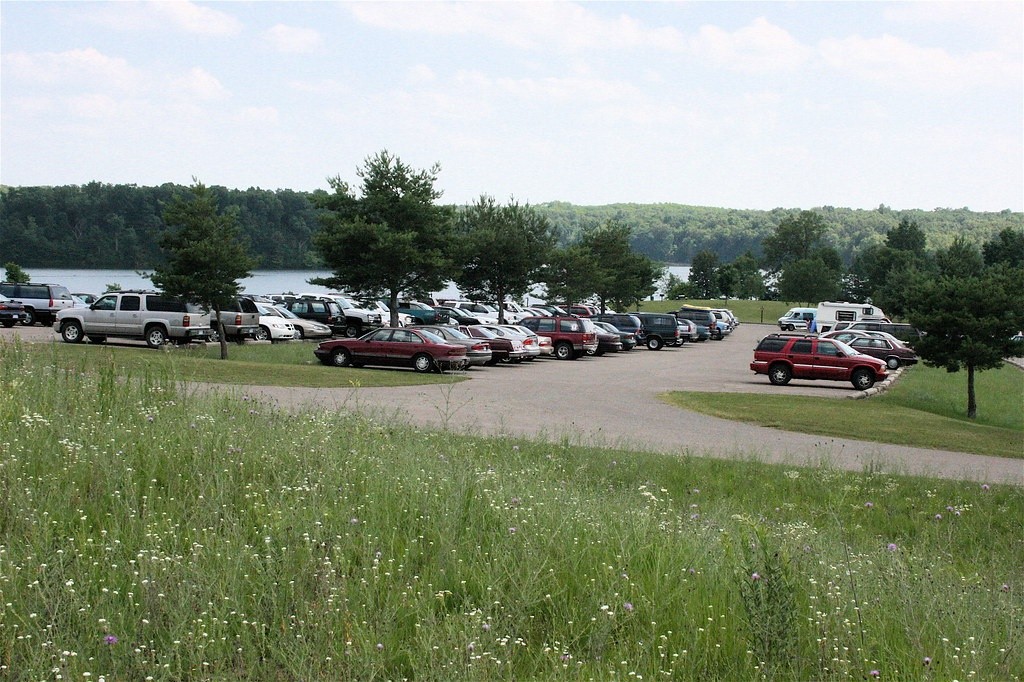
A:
[53,289,215,348]
[748,333,891,391]
[0,282,76,327]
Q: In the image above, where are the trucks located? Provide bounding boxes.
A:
[816,301,893,334]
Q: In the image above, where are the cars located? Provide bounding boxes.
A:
[313,326,471,373]
[410,324,493,370]
[819,318,925,349]
[837,336,918,371]
[0,294,27,329]
[70,285,741,364]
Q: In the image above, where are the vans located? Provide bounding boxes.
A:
[776,307,817,331]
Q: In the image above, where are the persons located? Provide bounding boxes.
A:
[806,317,817,332]
